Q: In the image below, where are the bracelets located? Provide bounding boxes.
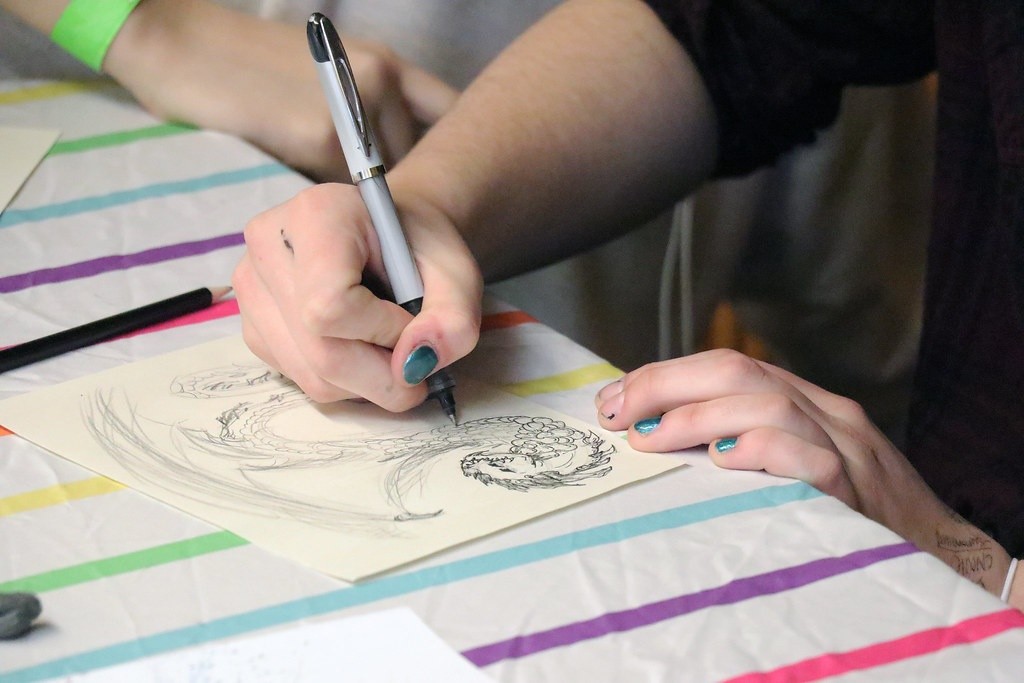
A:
[999,558,1018,603]
[50,0,141,71]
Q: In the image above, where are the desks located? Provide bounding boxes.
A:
[0,0,1024,683]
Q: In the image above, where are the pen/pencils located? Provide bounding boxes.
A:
[0,286,233,374]
[307,12,458,427]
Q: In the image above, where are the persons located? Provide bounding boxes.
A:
[232,0,1024,610]
[0,0,460,186]
